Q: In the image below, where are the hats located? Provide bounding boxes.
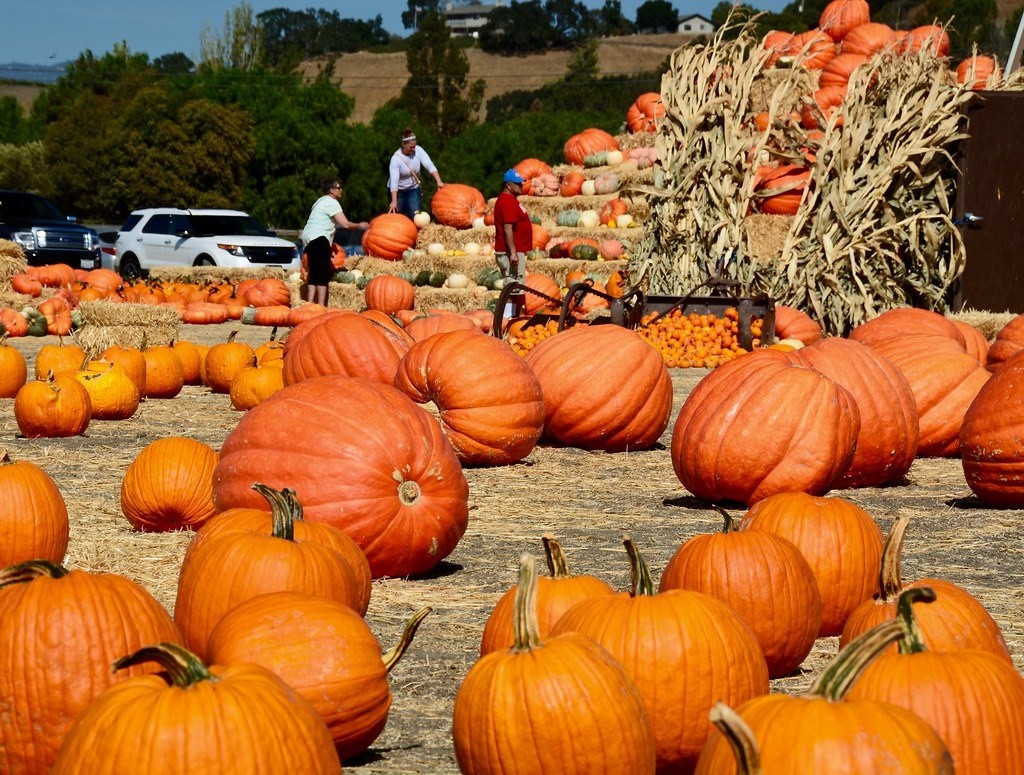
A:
[504,168,526,183]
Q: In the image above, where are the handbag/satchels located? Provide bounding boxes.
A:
[418,184,424,198]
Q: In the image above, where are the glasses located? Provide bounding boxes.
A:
[330,186,341,191]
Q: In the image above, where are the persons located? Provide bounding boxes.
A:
[494,169,532,316]
[302,177,370,306]
[387,128,448,222]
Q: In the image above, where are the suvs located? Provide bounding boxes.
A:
[92,203,367,286]
[0,190,102,270]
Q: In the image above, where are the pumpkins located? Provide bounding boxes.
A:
[0,0,1024,775]
